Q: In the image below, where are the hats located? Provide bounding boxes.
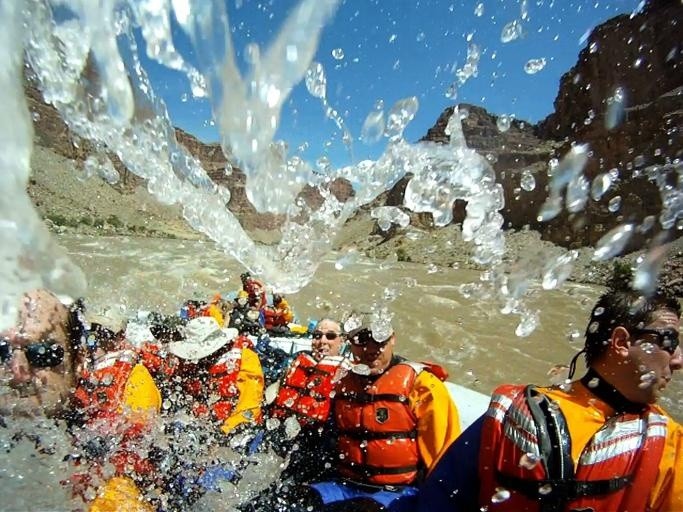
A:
[169,316,239,360]
[234,291,249,303]
[88,306,127,334]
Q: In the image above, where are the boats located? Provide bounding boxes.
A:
[73,322,495,512]
[173,283,313,340]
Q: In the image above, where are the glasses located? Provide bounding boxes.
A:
[629,327,680,356]
[312,333,343,340]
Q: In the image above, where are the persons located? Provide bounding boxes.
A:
[411,278,682,512]
[0,274,457,512]
